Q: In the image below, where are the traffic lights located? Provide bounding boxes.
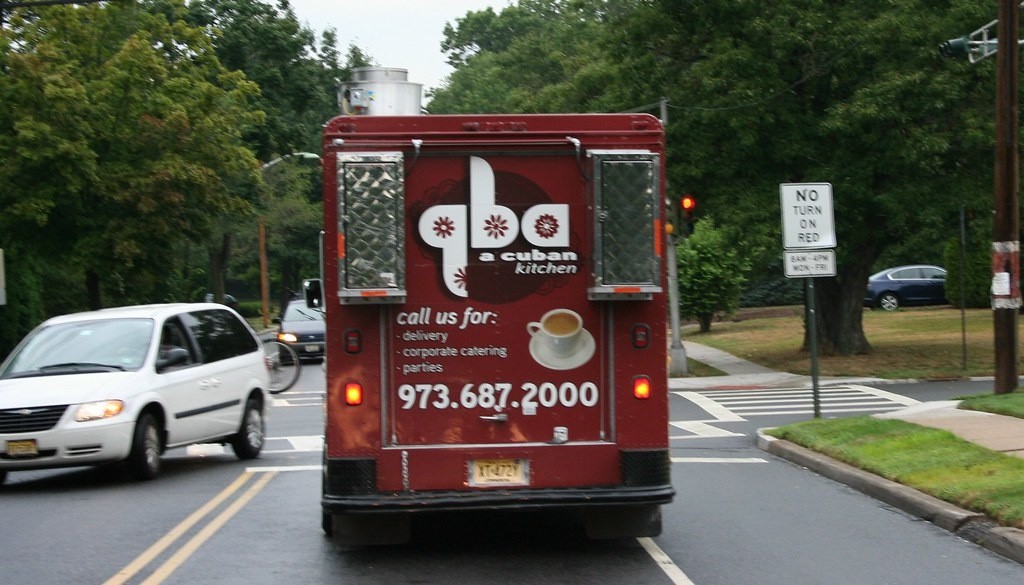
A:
[679,193,696,239]
[665,196,677,243]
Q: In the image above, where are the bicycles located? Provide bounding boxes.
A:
[260,339,301,394]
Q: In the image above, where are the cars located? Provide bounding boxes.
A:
[0,303,271,483]
[272,300,326,364]
[859,266,952,311]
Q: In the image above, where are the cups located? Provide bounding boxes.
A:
[526,309,583,358]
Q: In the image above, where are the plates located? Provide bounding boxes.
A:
[529,327,596,370]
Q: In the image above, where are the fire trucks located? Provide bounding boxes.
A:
[299,64,675,547]
